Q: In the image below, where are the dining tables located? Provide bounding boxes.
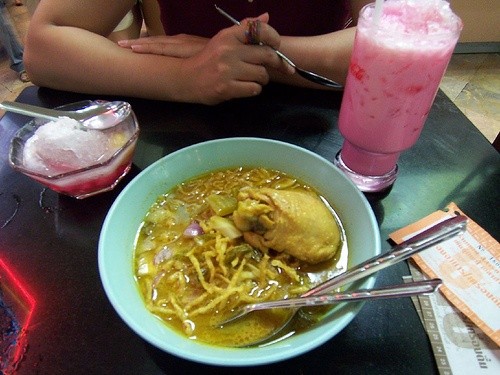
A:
[0,86,500,375]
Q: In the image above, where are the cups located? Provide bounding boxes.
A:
[333,0,463,192]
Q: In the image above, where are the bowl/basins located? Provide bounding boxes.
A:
[97,137,382,367]
[8,101,140,201]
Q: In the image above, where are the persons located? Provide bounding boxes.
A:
[0,0,31,82]
[23,0,374,104]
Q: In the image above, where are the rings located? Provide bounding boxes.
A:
[246,19,262,45]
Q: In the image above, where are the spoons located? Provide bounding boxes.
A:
[0,99,132,132]
[232,214,468,347]
[213,4,346,88]
